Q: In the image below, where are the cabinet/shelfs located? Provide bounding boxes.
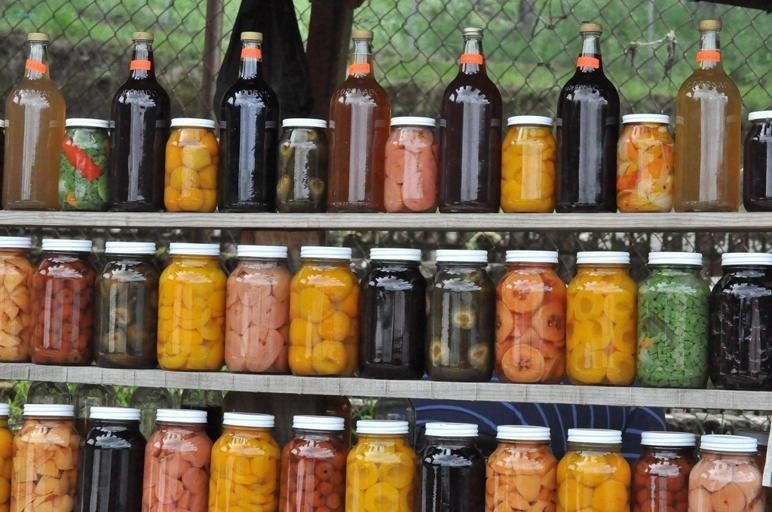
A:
[1,209,772,412]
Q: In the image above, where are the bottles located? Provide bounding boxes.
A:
[215,31,281,213]
[1,33,66,210]
[326,28,392,213]
[109,31,170,213]
[556,21,620,213]
[438,27,504,213]
[675,18,741,213]
[735,432,769,474]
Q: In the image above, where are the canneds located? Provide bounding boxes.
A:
[10,405,81,512]
[495,249,568,385]
[385,116,438,213]
[57,117,109,212]
[165,117,219,213]
[90,241,160,369]
[0,403,14,512]
[423,249,497,384]
[616,114,674,213]
[555,428,632,512]
[79,407,148,512]
[278,416,350,511]
[33,239,97,365]
[562,251,638,387]
[345,419,421,511]
[712,252,772,390]
[743,111,772,212]
[636,252,713,390]
[688,434,772,511]
[285,245,360,379]
[486,425,560,511]
[416,421,487,511]
[142,409,212,511]
[631,431,699,511]
[359,247,429,380]
[501,116,556,213]
[275,119,327,212]
[0,236,35,363]
[224,244,291,376]
[205,413,283,511]
[155,242,227,372]
